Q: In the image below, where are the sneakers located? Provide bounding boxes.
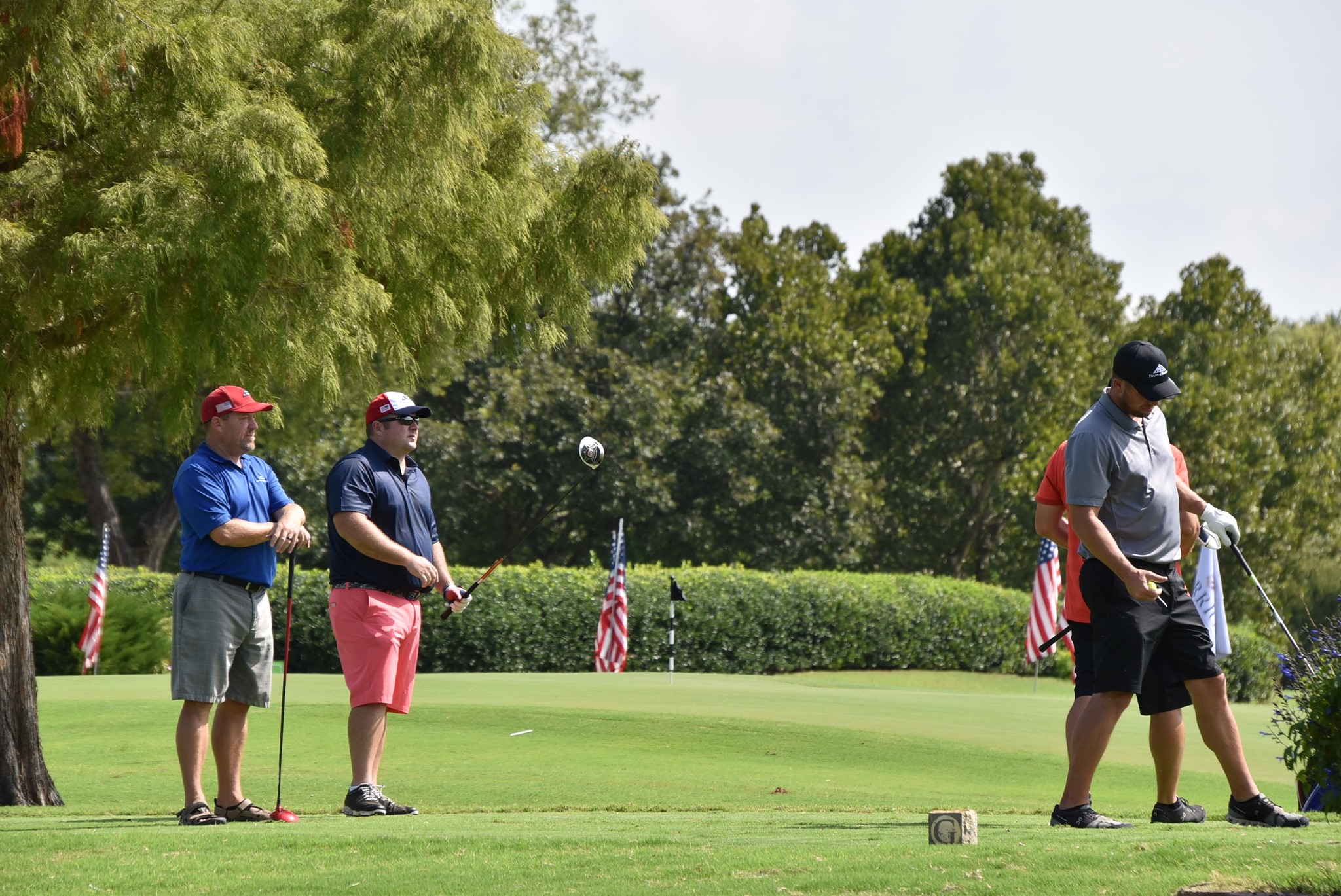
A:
[342,784,386,816]
[1088,794,1092,808]
[1049,803,1134,830]
[372,786,419,815]
[1150,795,1206,823]
[1225,793,1309,829]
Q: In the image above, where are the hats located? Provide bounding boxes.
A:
[200,386,273,423]
[1113,341,1182,401]
[365,391,431,426]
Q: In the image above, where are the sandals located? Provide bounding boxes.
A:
[213,798,273,822]
[176,802,226,825]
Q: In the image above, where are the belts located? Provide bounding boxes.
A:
[180,570,263,593]
[331,582,421,601]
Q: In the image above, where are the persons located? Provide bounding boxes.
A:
[324,391,475,819]
[1034,341,1309,829]
[170,385,311,825]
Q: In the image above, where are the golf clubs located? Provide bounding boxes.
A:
[1228,532,1319,676]
[438,436,605,622]
[268,546,299,823]
[1038,520,1222,653]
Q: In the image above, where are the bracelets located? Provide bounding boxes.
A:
[441,583,458,602]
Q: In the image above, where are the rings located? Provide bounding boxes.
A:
[286,537,292,541]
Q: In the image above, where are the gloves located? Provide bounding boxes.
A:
[1200,502,1240,546]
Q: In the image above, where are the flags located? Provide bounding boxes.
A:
[1058,596,1076,680]
[1188,522,1233,664]
[77,524,109,676]
[593,526,628,672]
[1025,537,1062,665]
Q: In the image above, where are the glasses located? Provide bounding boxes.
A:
[378,415,420,426]
